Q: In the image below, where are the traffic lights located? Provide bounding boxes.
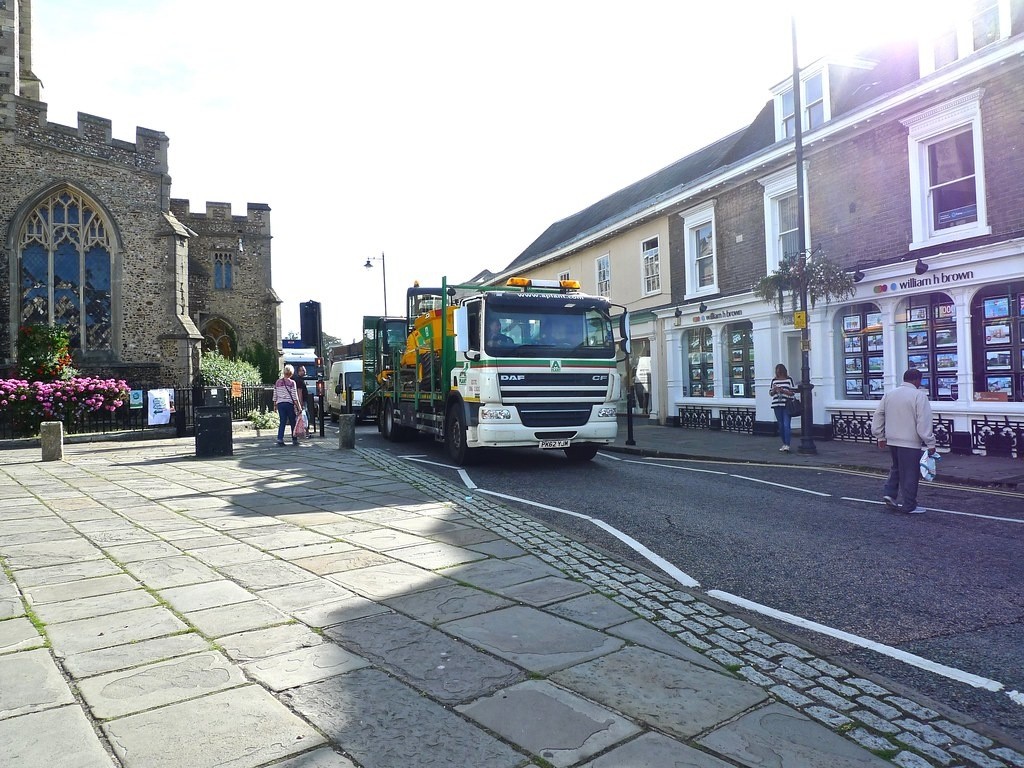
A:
[315,357,324,397]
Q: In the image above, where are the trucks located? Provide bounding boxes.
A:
[283,347,327,415]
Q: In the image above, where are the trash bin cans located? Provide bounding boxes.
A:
[194,404,234,459]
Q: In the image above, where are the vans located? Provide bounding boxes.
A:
[326,359,378,423]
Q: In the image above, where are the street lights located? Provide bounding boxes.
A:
[365,252,387,316]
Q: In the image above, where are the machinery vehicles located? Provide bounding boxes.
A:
[361,276,632,467]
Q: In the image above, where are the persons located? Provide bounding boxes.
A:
[486,317,514,348]
[769,364,801,452]
[273,364,314,446]
[871,369,940,513]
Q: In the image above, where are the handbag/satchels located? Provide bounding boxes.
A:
[302,410,308,428]
[293,413,305,437]
[919,450,942,483]
[786,390,802,416]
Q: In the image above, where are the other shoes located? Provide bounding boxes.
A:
[883,496,898,508]
[907,506,926,514]
[277,441,287,447]
[292,441,301,446]
[779,445,790,452]
[306,435,311,439]
